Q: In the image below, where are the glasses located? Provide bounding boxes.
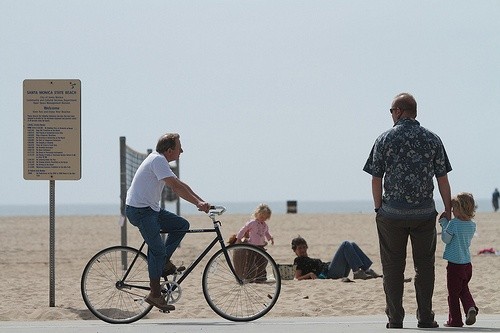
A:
[389,108,399,113]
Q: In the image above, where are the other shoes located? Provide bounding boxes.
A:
[249,277,268,284]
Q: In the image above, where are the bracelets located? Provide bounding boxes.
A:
[374,208,379,213]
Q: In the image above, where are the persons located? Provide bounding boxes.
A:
[437,193,478,327]
[125,134,210,312]
[291,235,383,280]
[492,188,499,211]
[237,203,274,282]
[362,93,453,327]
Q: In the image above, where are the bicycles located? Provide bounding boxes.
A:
[81,206,281,324]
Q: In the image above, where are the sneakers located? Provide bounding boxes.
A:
[145,291,175,311]
[417,320,439,328]
[466,306,477,325]
[162,262,186,278]
[443,322,464,327]
[354,269,379,280]
[386,320,403,329]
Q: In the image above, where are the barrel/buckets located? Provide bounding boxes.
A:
[273,264,297,280]
